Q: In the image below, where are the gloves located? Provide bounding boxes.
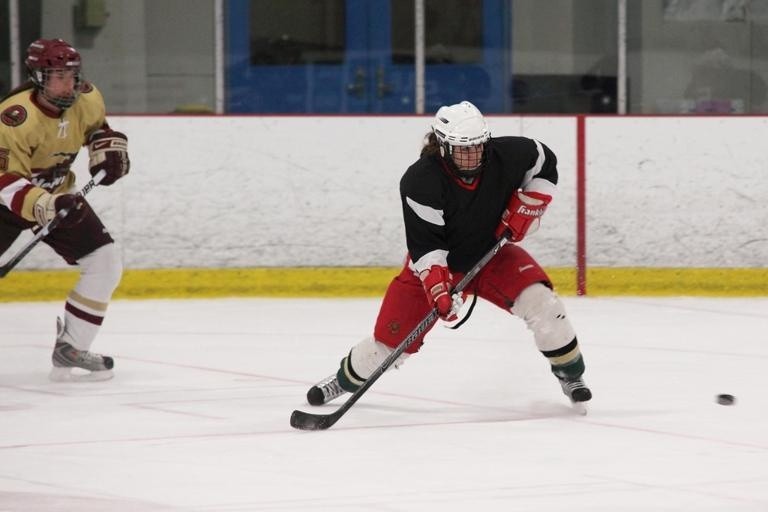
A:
[33,189,90,236]
[86,130,131,187]
[416,265,470,324]
[497,187,553,240]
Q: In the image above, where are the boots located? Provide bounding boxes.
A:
[51,317,115,372]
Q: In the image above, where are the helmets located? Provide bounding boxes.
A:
[24,37,85,109]
[431,98,496,183]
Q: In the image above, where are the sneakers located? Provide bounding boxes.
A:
[558,378,593,403]
[307,373,349,407]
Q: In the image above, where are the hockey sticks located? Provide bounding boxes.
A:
[290,229,513,430]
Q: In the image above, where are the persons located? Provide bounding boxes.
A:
[1,38,130,371]
[305,98,593,407]
